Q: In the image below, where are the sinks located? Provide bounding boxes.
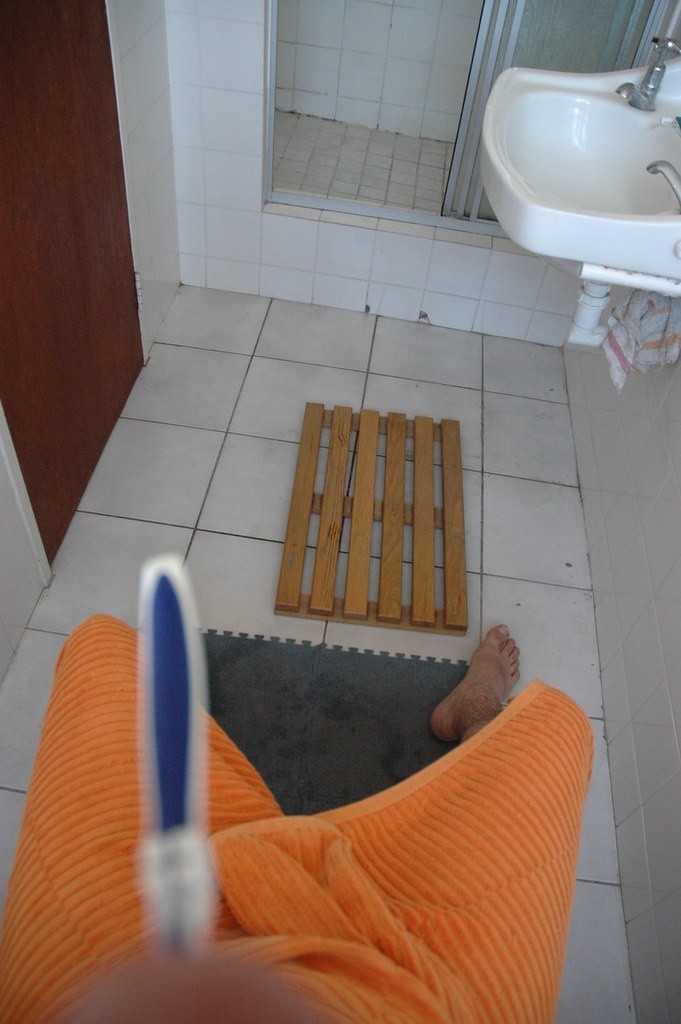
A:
[480,58,677,284]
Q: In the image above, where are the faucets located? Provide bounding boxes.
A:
[646,160,681,204]
[614,37,681,112]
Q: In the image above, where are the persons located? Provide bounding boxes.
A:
[0,614,598,1024]
